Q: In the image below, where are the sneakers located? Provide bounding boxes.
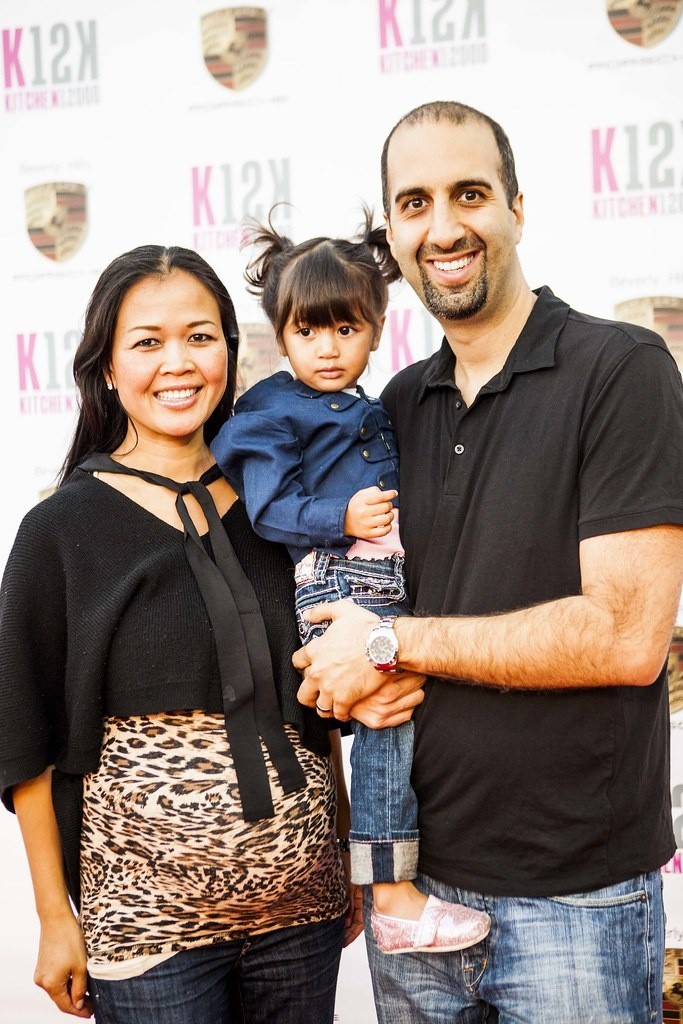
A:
[370,892,493,955]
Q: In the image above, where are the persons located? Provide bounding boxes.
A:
[209,199,492,957]
[2,244,363,1024]
[293,102,681,1023]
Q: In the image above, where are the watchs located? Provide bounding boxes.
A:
[364,616,406,675]
